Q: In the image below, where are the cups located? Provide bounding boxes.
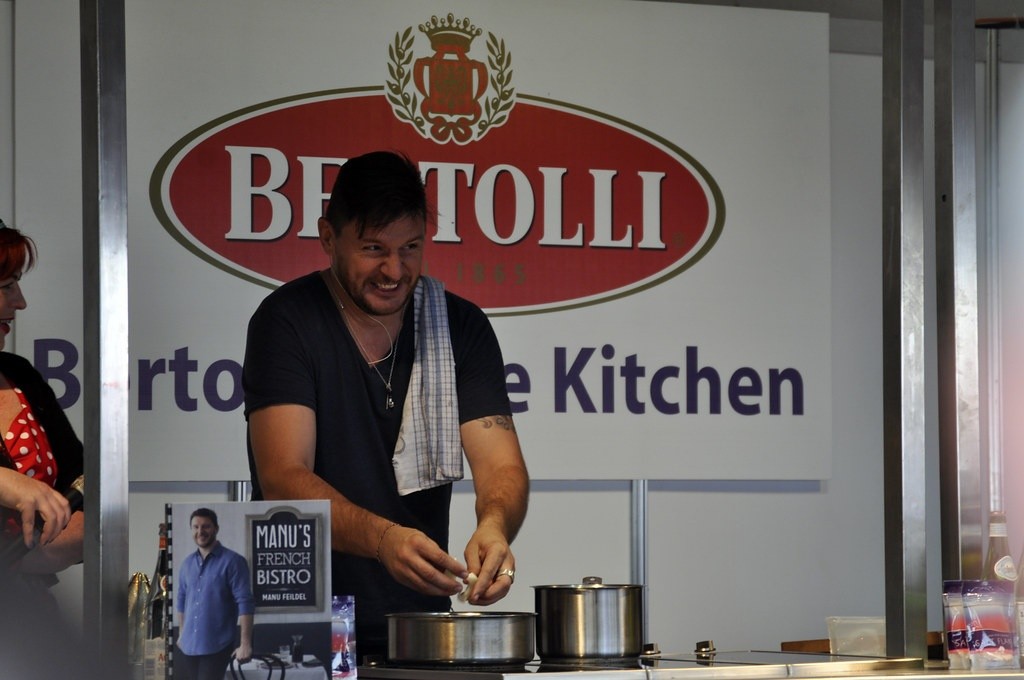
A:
[279,645,290,660]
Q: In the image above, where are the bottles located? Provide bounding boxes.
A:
[980,510,1024,671]
[144,523,167,639]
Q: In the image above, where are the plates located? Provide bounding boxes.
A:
[260,662,293,669]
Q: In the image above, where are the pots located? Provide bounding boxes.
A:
[384,612,539,665]
[530,576,649,659]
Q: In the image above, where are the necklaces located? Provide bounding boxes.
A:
[326,267,412,409]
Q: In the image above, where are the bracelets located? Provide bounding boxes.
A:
[376,522,400,565]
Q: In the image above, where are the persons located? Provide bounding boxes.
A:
[172,507,254,679]
[239,151,528,663]
[0,218,85,622]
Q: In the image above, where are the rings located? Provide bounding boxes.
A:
[496,568,515,584]
